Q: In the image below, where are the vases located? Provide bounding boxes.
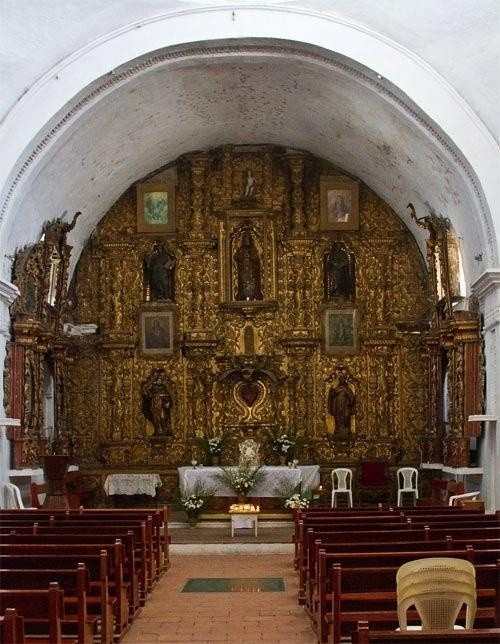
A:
[186,514,199,527]
[235,492,250,503]
[209,454,220,466]
[278,454,286,465]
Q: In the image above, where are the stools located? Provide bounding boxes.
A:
[226,501,262,539]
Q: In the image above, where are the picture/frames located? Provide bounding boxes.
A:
[135,180,179,234]
[316,175,361,232]
[323,306,361,356]
[137,301,178,359]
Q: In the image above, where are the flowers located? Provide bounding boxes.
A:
[190,423,234,455]
[278,482,310,508]
[263,424,305,455]
[172,479,216,515]
[211,460,268,493]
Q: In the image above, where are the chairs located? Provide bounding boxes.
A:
[330,468,354,508]
[395,558,478,633]
[447,491,481,509]
[395,467,420,509]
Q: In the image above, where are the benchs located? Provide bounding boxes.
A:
[0,504,174,644]
[294,507,500,644]
[31,469,88,510]
